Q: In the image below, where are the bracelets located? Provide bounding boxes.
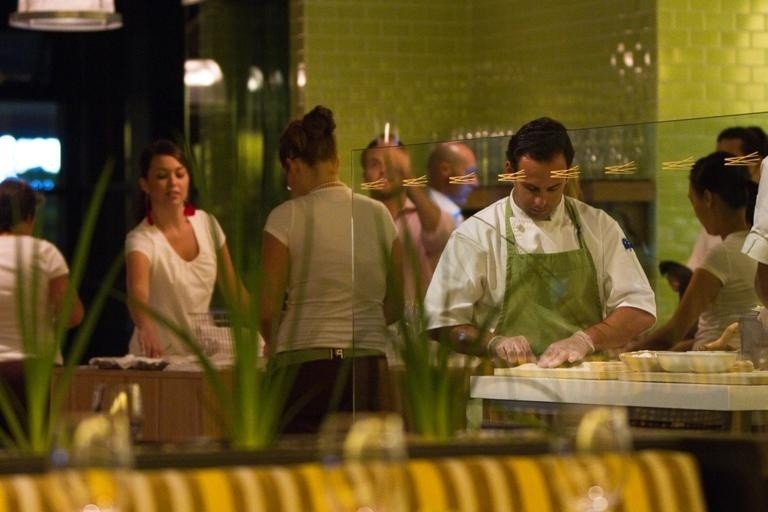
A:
[572,329,595,355]
[485,335,507,361]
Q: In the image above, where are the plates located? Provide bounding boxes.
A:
[96,358,167,371]
[584,361,621,371]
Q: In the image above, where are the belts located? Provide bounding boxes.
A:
[274,347,384,372]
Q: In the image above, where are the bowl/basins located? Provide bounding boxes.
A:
[619,351,739,373]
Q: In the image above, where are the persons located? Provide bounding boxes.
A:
[120,139,257,366]
[738,153,767,311]
[427,139,477,227]
[0,176,88,435]
[354,131,456,362]
[422,117,657,372]
[670,122,767,279]
[250,103,411,448]
[616,151,756,353]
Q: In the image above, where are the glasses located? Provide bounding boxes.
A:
[284,155,299,192]
